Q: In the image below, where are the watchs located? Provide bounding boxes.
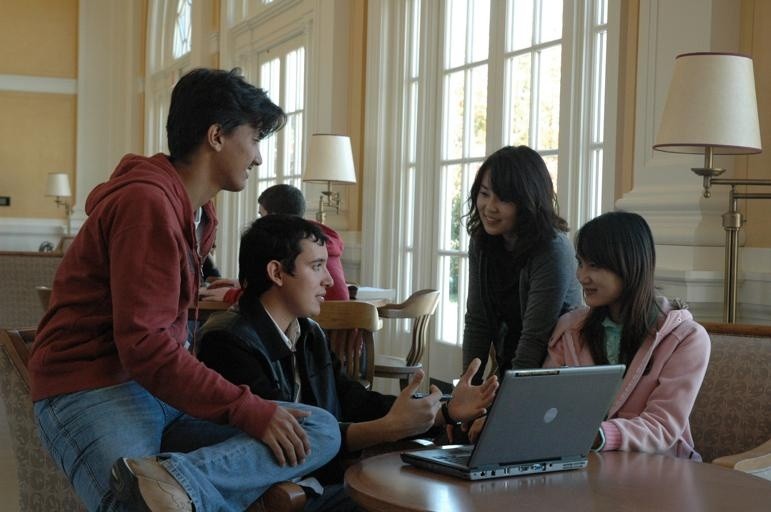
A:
[441,402,463,427]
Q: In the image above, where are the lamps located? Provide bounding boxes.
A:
[43,171,75,236]
[302,133,358,226]
[652,52,770,325]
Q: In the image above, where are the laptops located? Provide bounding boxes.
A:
[399,362,627,481]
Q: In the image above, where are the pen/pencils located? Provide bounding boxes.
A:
[414,393,452,398]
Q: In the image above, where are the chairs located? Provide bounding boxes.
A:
[1,326,89,510]
[1,253,442,395]
[687,326,770,481]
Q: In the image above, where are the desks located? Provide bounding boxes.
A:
[345,443,768,512]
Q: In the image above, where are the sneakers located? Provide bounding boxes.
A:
[109,455,194,512]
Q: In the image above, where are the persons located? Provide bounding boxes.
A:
[466,210,712,463]
[199,251,222,282]
[26,66,342,512]
[196,213,499,511]
[443,146,587,445]
[197,184,364,366]
